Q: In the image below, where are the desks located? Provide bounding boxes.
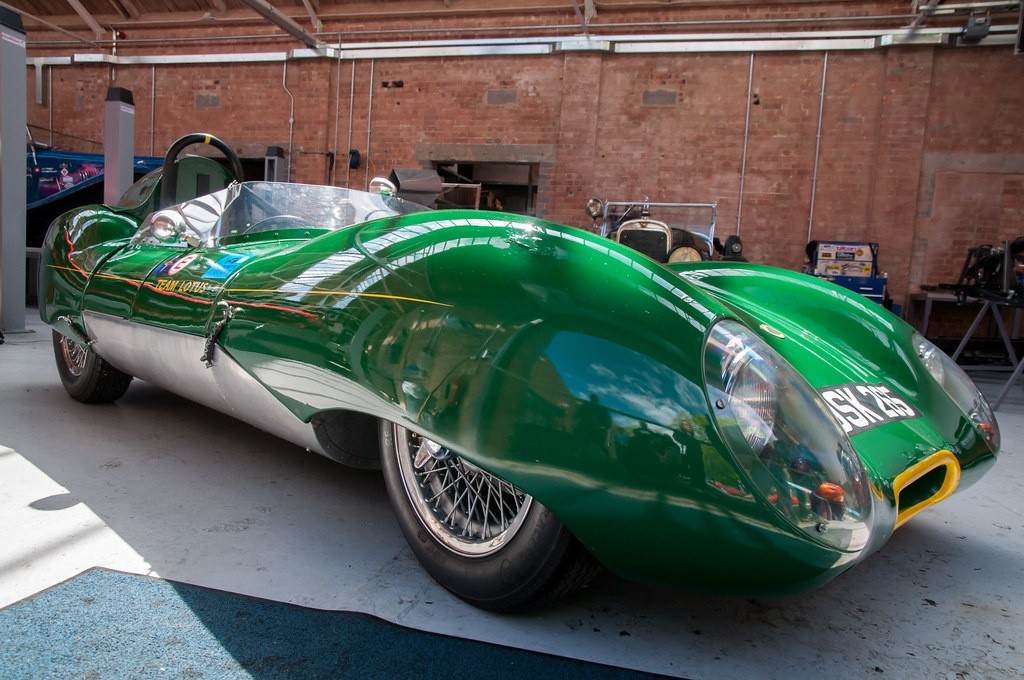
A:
[907,292,1024,371]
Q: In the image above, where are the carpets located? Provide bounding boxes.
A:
[0,566,686,680]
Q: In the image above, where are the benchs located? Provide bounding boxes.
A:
[603,229,713,258]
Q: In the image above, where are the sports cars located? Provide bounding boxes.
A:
[36,130,1001,617]
